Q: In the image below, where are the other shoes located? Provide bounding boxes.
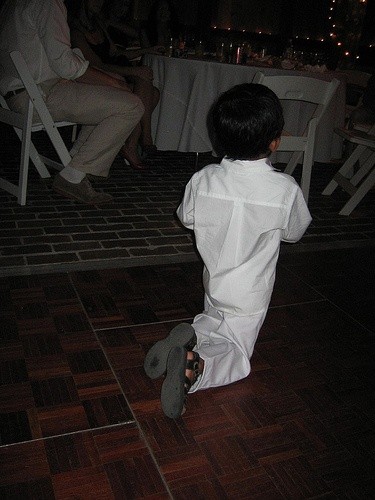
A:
[52,172,114,204]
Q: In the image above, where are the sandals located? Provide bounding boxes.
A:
[158,345,202,419]
[143,323,199,380]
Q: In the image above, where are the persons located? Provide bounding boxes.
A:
[144,83,313,421]
[0,0,146,204]
[345,71,375,158]
[103,0,139,44]
[70,0,165,171]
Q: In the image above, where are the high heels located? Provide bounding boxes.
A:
[119,144,149,170]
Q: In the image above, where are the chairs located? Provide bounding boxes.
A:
[322,124,375,215]
[0,49,80,206]
[343,69,372,129]
[251,72,340,203]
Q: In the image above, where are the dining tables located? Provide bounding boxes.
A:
[142,52,347,169]
